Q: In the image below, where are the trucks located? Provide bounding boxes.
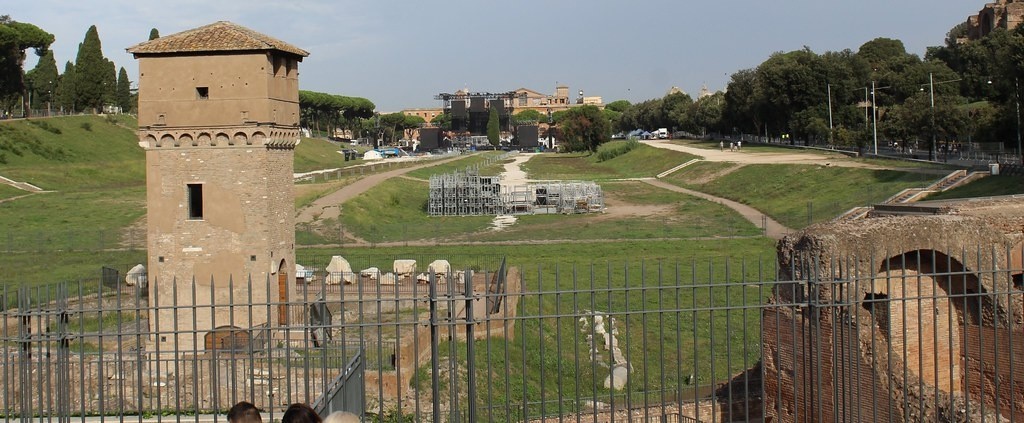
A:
[658,128,668,139]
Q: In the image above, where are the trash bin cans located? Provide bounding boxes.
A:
[345,153,355,160]
[990,164,999,175]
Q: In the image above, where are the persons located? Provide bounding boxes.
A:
[720,141,724,152]
[281,403,361,423]
[227,401,263,423]
[889,141,958,157]
[730,141,742,152]
